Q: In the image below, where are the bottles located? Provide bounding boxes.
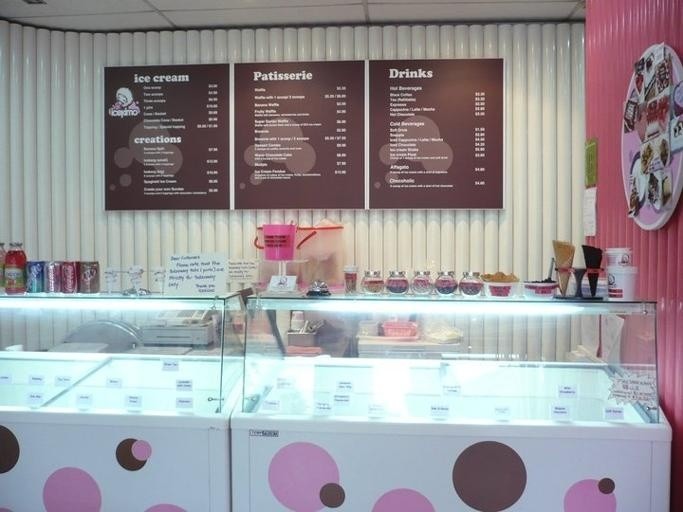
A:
[0,242,26,294]
[342,266,357,296]
[459,271,483,298]
[360,270,385,295]
[385,270,409,295]
[410,270,434,296]
[434,270,458,296]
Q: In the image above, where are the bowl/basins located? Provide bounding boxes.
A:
[522,282,558,301]
[483,281,520,298]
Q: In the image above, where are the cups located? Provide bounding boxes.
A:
[291,311,305,331]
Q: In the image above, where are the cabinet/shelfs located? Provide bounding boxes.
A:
[229,292,674,512]
[0,291,247,512]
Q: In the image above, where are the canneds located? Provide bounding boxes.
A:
[79,262,99,294]
[42,262,61,292]
[59,261,78,294]
[24,259,44,293]
